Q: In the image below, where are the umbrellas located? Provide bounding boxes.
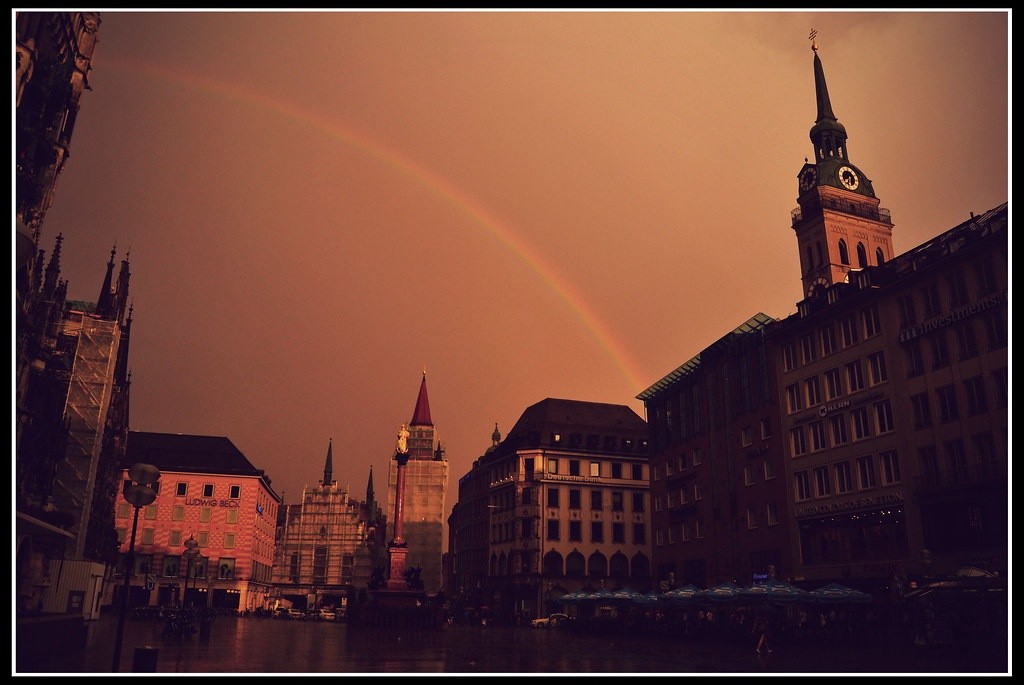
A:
[558,568,1008,632]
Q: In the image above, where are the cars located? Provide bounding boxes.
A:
[530,613,574,628]
[274,608,285,618]
[282,609,301,619]
[310,609,336,621]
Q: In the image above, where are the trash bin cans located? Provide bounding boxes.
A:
[199,620,211,644]
[130,645,160,672]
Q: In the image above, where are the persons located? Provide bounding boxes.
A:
[681,608,715,636]
[751,607,773,654]
[799,608,837,634]
